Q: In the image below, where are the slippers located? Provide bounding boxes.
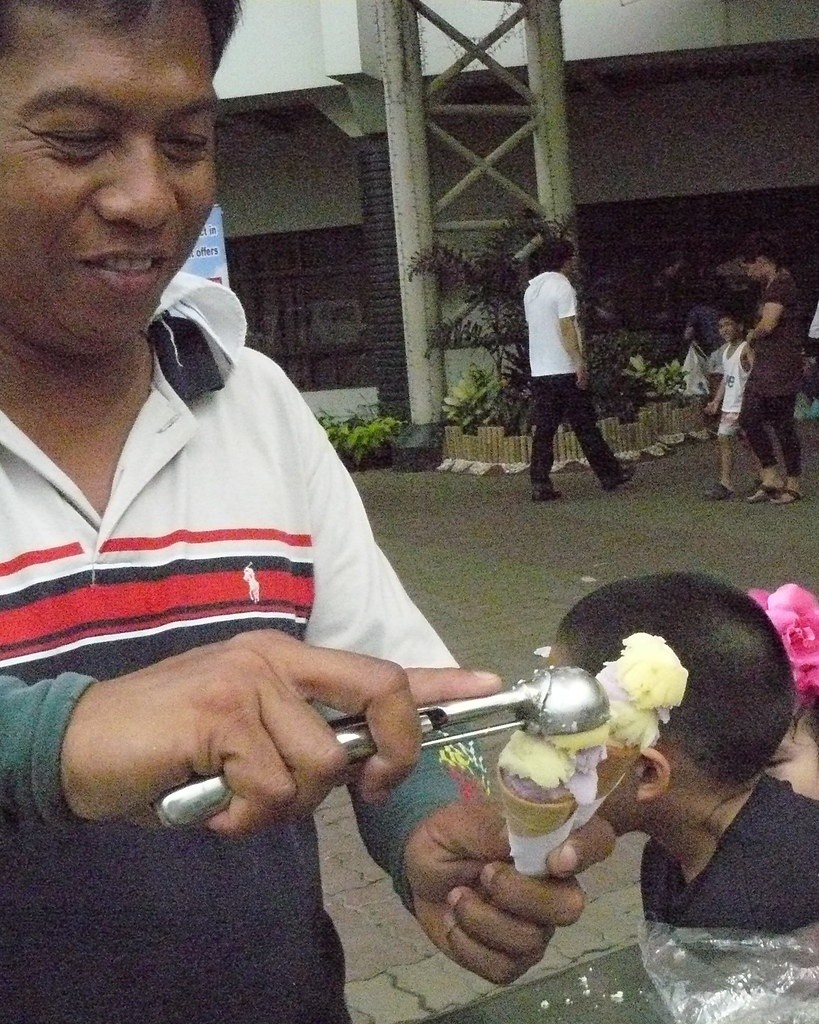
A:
[745,485,805,506]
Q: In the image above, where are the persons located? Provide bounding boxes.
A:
[748,583,819,799]
[0,0,617,1024]
[524,239,636,501]
[684,239,819,503]
[547,572,819,1024]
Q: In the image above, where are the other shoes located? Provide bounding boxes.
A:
[601,467,637,492]
[706,483,734,499]
[532,485,562,501]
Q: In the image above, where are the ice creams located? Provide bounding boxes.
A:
[496,629,688,839]
